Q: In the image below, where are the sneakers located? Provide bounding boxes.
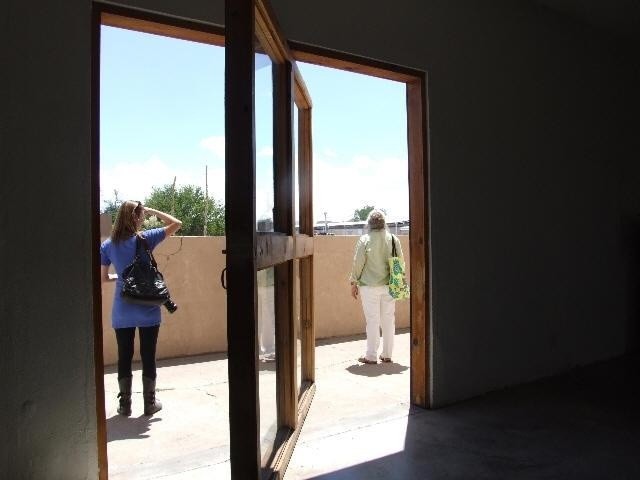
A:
[358,356,377,364]
[379,354,391,362]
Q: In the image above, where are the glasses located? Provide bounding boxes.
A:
[130,201,141,219]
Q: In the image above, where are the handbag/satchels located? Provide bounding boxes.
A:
[388,234,410,301]
[120,233,170,307]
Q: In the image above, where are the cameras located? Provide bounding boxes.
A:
[165,299,178,313]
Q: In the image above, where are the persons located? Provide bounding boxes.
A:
[100,199,183,419]
[350,209,407,365]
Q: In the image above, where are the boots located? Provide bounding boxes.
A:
[116,375,133,416]
[141,374,162,415]
